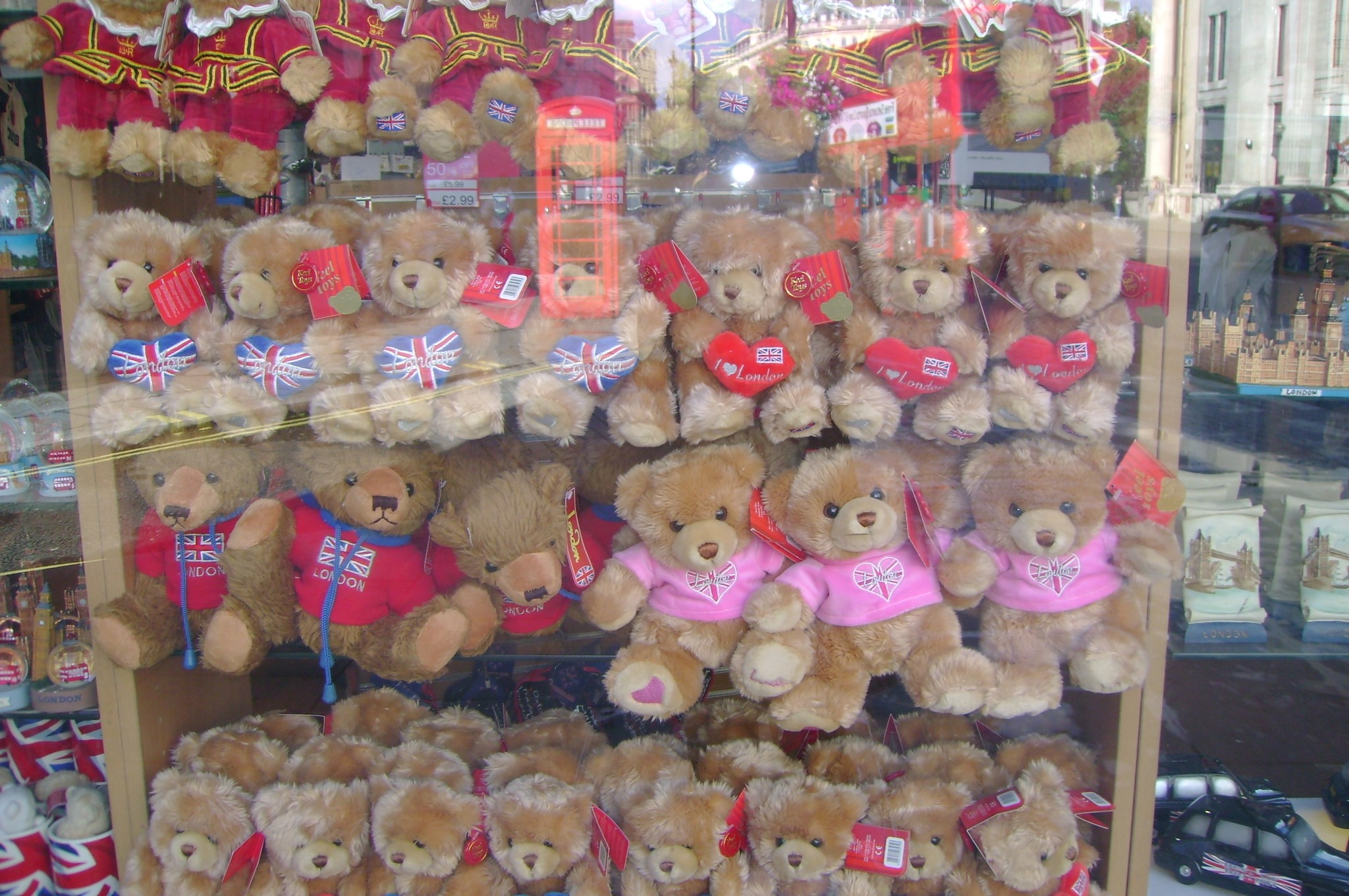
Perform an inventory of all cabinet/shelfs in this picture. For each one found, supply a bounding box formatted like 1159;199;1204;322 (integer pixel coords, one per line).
1167;107;1348;661
0;64;99;721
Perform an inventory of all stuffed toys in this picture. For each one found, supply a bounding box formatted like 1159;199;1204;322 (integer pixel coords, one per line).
226;438;463;682
570;439;817;721
940;438;1150;724
63;202;290;445
660;204;833;441
982;198;1148;435
307;207;531;448
0;0;1175;895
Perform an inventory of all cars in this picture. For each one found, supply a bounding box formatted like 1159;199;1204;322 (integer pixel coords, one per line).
1199;183;1349;271
1154;753;1286;846
1153;793;1349;895
